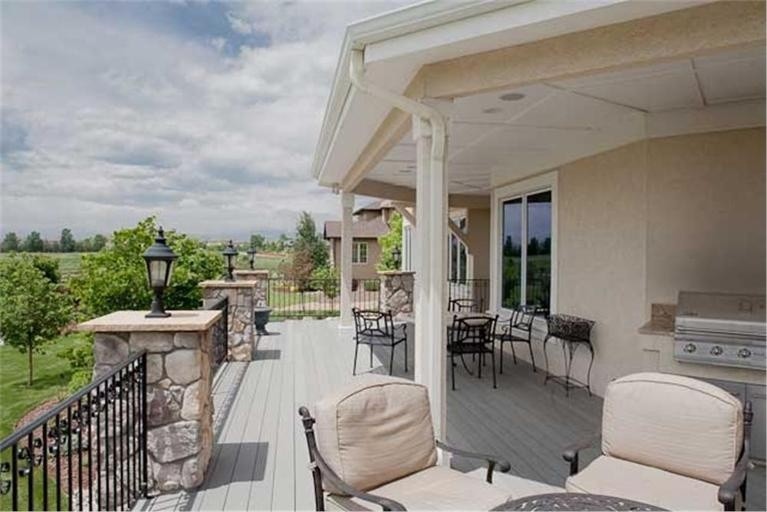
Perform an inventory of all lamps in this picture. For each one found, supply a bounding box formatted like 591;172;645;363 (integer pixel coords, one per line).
392;245;402;270
223;238;256;282
140;226;177;318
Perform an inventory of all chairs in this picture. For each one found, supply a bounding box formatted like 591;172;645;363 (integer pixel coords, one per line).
447;296;537;391
297;374;514;510
351;307;407;377
559;370;754;506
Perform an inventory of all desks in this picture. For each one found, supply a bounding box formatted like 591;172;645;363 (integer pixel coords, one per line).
491;490;669;510
